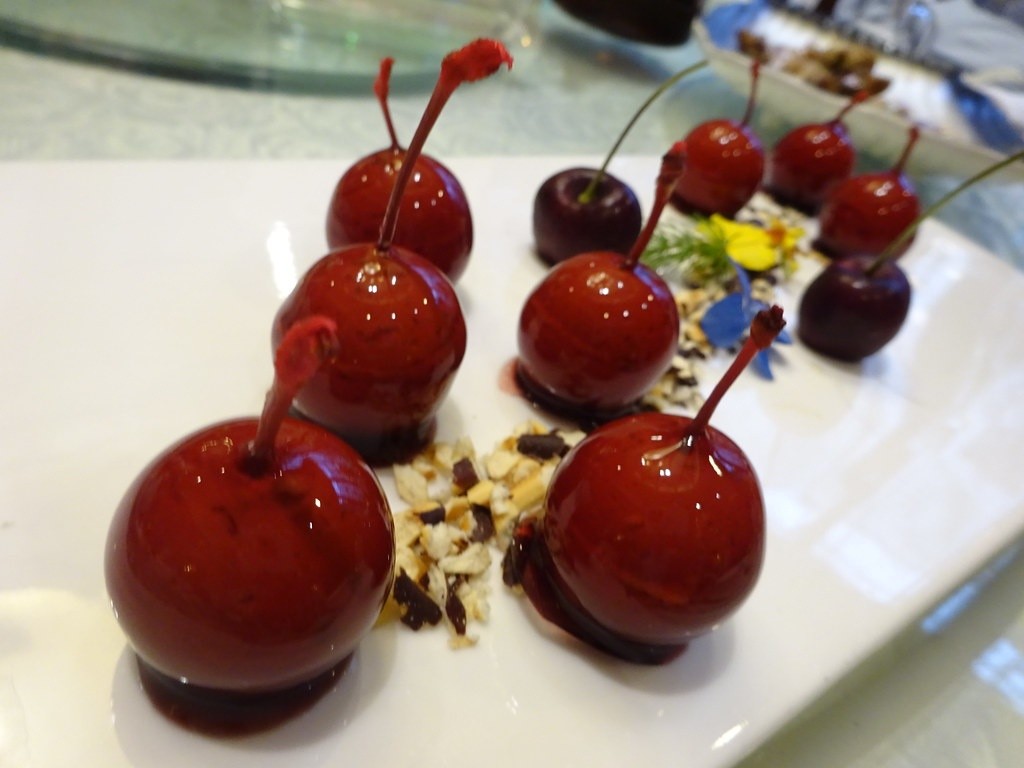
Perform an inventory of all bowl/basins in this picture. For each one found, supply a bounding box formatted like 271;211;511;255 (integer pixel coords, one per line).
692;11;1022;188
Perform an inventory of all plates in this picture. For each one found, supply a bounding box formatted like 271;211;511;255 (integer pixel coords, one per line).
3;149;1024;767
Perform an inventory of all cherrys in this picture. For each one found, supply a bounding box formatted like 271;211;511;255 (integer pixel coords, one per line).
103;29;1021;689
